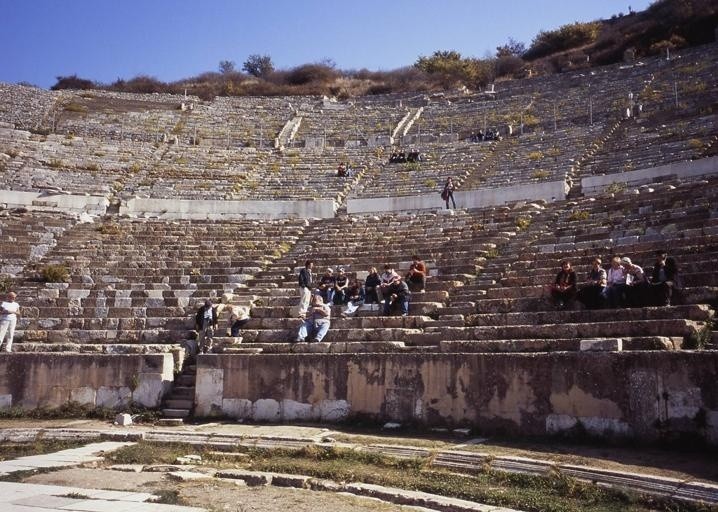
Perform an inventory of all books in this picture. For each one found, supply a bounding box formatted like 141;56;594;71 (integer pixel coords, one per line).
626;273;634;285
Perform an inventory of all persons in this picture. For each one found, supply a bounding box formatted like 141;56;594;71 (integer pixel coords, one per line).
445;177;456;209
621;257;647;309
293;254;427;343
225;304;250;337
577;257;607;310
646;249;683;306
601;256;627;308
0;292;20;353
196;300;218;354
338;161;353;177
390;148;425;162
465;127;500;142
550;260;579;310
